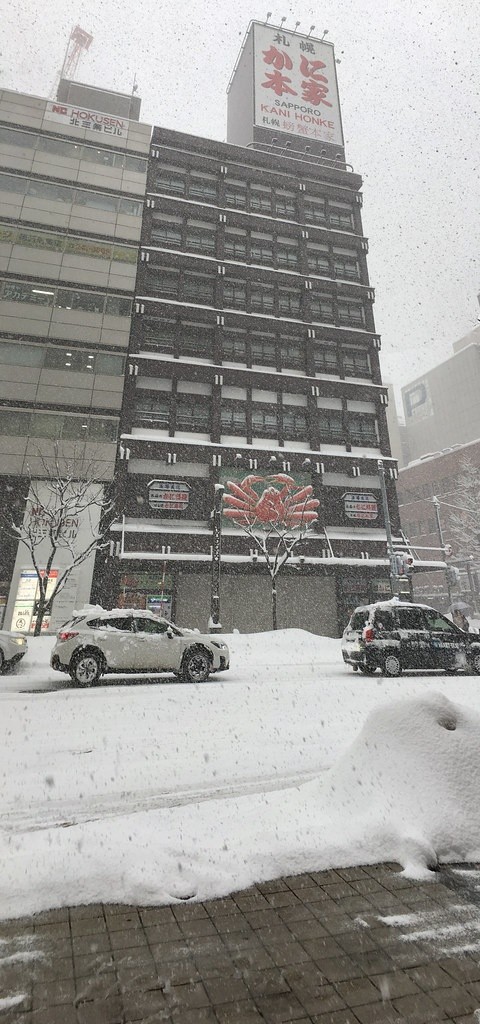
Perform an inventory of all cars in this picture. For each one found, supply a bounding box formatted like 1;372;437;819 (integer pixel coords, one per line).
0;628;28;676
342;601;480;677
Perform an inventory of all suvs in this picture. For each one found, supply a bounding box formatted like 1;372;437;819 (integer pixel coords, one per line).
49;608;231;688
